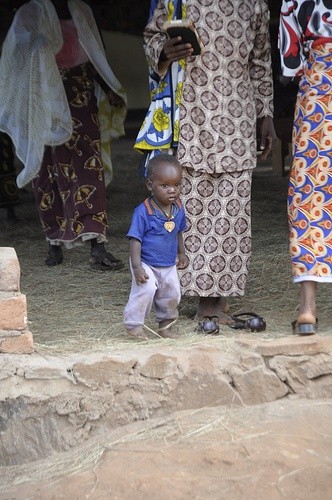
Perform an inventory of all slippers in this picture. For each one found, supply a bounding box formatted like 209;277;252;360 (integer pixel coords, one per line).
291;317;319;335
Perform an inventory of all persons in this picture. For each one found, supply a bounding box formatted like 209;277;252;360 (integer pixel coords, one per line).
122;154;189;338
278;0;332;334
0;0;125;272
144;0;277;325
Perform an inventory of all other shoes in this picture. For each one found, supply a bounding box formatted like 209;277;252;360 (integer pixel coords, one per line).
7;216;21;225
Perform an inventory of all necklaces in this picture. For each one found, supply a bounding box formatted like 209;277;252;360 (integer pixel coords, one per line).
151;196;175;233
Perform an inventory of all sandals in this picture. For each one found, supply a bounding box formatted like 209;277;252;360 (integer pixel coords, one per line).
199;316;219;334
89;252;122;270
46;248;63;265
229;312;265;331
